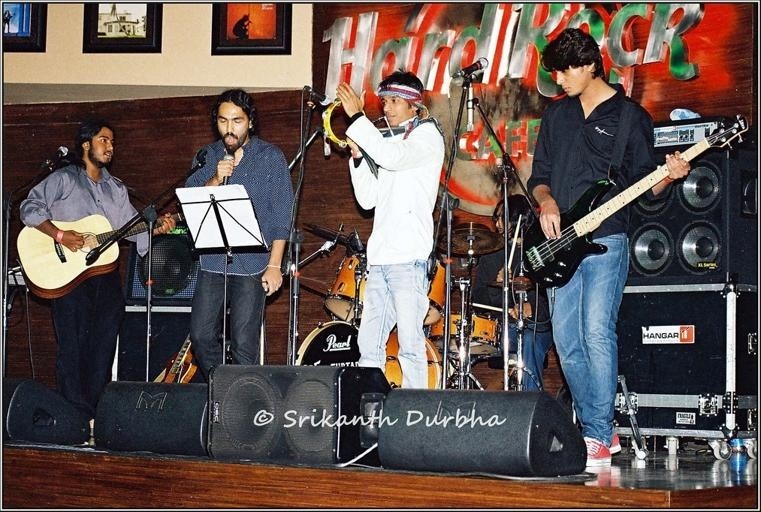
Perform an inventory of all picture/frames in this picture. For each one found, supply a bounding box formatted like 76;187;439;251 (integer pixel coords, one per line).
82;2;162;53
2;1;48;52
211;2;292;55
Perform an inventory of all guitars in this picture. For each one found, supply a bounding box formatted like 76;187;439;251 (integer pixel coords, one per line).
154;331;197;385
17;211;185;299
523;113;748;289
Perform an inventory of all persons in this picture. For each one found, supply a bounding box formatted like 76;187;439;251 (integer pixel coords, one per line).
333;70;445;389
471;192;553;393
527;27;692;469
182;86;294;382
19;116;177;454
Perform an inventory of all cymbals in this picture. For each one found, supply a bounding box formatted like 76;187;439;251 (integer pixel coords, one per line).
302;219;348;244
437;227;505;254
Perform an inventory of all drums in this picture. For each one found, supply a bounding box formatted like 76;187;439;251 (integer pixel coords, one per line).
430;308;502;352
325;254;367;320
297;318;443;389
425;260;450;328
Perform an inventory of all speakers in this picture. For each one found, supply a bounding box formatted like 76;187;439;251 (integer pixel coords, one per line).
2;377;91;446
117;235;206;382
378;388;587;477
624;115;758;284
94;380;208;457
207;365;390;468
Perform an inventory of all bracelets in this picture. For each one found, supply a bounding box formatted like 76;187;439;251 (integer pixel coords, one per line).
664;176;673;185
268;264;282;269
56;230;64;243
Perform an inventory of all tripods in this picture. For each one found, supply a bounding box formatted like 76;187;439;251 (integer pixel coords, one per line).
507;225;542;391
445;281;483;391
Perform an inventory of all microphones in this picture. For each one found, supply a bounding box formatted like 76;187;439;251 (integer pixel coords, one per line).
453;57;488;80
222;154;235;185
354;227;364;251
303;85;331;107
40;145;69;168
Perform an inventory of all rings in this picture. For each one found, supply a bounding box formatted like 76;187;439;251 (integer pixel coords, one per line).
261;281;266;283
684;161;687;166
73;247;75;250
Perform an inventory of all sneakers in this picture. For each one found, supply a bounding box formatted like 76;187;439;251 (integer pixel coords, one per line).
584;430;622;467
